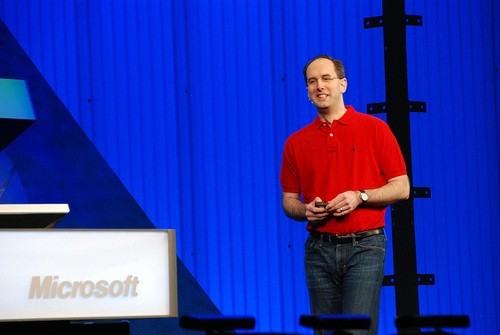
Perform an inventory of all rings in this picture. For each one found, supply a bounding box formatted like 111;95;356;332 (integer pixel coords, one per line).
340;208;342;211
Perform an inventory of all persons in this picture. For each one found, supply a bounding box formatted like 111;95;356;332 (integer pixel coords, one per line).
279;55;410;335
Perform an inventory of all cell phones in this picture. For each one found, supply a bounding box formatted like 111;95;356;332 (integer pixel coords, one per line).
314;202;328;215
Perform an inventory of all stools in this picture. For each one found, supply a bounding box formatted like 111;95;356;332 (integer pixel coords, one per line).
179;314;256;335
299;314;372;335
394;314;470;335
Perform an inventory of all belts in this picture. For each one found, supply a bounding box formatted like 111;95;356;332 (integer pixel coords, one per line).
309;229;383;243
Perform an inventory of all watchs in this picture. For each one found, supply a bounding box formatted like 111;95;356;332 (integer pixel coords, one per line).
359;189;368;205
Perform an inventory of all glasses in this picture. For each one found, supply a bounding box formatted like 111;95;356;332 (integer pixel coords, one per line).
306;75;339;85
